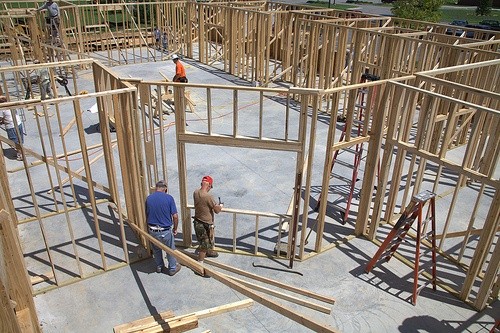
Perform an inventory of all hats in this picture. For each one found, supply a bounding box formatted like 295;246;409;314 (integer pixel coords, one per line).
171;53;180;60
202;176;213;188
156;180;167;192
0;95;7;103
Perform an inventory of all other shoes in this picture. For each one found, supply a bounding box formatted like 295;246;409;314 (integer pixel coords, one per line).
156;265;165;273
17;154;22;161
207;251;219;257
169;264;181;276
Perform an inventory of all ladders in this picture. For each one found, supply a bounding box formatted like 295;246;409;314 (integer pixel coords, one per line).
44;16;78;79
365;190;436;304
489;320;500;333
315;73;381;225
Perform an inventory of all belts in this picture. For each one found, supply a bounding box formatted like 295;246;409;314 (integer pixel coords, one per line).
147;227;169;232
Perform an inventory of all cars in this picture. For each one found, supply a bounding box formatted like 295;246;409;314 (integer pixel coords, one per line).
479;19;500;27
446;19;468;34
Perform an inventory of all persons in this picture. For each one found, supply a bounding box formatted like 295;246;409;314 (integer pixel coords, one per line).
30;0;62;37
192;175;224;278
33;58;55;106
145;182;182;276
171;54;189;83
0;96;26;160
155;24;169;51
